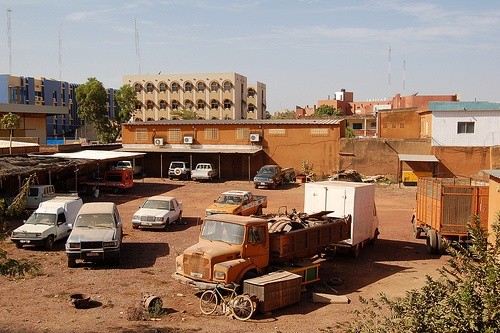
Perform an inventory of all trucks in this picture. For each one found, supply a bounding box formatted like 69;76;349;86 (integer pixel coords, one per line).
64;166;134;194
304;180;380;257
171;215;351;293
9;196;84;250
410;176;489;254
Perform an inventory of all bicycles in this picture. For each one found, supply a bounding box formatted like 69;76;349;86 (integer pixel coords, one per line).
200;281;254;321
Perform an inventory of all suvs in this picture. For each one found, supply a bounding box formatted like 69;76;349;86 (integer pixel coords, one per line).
168;161;190;180
253;165;284;190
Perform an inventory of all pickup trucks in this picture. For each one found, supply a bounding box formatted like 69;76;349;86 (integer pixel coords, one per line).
4;184;78;212
205;191;268;217
110;161;143;176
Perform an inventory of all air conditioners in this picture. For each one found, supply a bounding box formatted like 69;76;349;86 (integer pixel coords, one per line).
249;133;259;142
184;136;193;144
154;138;163;146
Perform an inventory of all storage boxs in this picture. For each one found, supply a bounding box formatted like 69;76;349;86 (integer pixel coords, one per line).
243;271;303;313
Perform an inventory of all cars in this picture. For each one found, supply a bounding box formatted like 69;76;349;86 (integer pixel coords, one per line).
191;163;219;181
131;196;182;231
65;202;123;267
